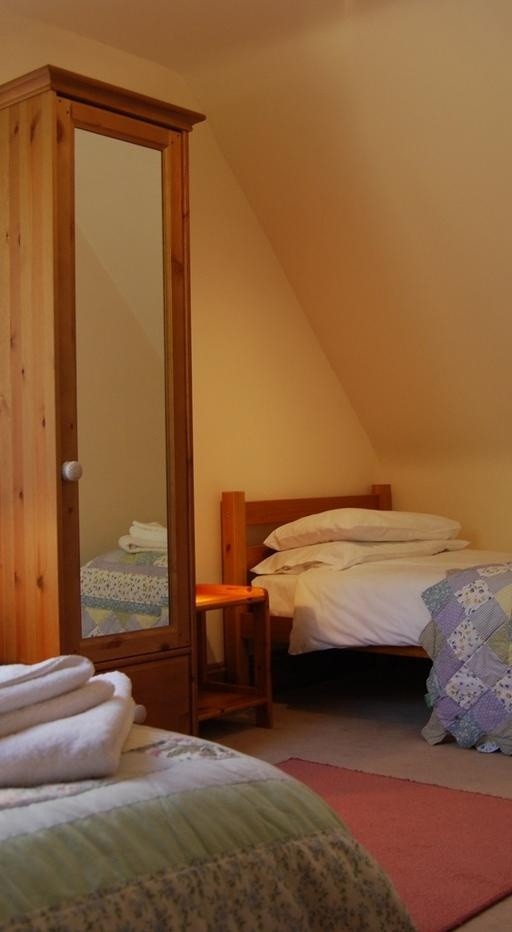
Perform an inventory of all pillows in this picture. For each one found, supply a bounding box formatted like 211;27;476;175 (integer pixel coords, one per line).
249;537;471;576
263;503;461;553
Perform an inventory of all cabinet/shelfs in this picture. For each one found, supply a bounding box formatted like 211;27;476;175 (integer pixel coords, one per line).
1;64;208;737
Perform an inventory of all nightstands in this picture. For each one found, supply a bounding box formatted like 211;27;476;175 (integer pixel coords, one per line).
197;584;275;732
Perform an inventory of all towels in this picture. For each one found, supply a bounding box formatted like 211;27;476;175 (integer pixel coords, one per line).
1;654;135;787
118;520;168;553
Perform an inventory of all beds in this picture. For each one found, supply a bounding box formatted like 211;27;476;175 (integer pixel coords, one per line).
81;547;171;640
222;481;511;758
1;715;414;931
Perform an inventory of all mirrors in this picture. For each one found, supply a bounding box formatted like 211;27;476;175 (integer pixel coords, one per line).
72;122;173;641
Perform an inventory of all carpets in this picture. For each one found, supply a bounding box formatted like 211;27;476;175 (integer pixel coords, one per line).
268;758;511;930
